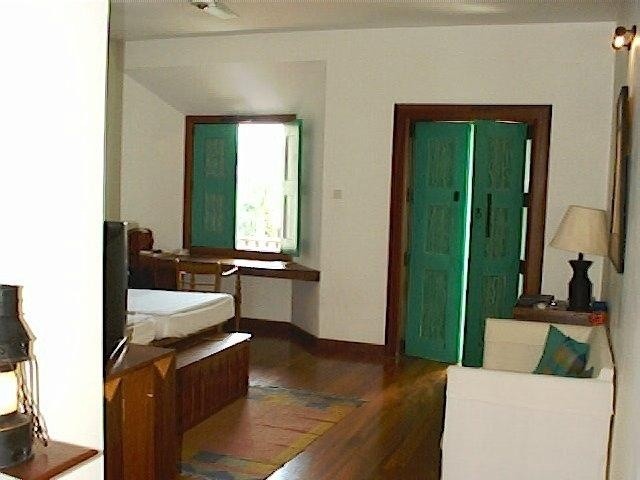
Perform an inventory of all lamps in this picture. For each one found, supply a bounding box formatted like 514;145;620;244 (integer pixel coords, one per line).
549;205;612;311
612;24;636;51
0;284;48;472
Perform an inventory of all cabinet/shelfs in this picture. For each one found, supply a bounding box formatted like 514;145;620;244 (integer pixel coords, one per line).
176;332;251;434
105;343;180;480
514;294;610;325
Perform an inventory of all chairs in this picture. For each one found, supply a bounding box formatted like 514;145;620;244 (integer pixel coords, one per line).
174;256;241;333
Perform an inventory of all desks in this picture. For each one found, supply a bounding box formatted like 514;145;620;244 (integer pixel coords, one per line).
155;254;319;281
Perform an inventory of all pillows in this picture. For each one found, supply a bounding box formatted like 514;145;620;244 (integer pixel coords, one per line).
532;324;590;378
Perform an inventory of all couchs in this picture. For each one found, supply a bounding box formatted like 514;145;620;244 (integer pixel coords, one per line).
440;317;614;480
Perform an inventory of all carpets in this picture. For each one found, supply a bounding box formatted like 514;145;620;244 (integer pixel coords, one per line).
180;383;365;480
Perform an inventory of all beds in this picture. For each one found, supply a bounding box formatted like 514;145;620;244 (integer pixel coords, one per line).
127;288;236;353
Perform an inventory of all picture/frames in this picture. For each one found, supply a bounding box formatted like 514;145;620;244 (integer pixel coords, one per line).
609;85;628;273
